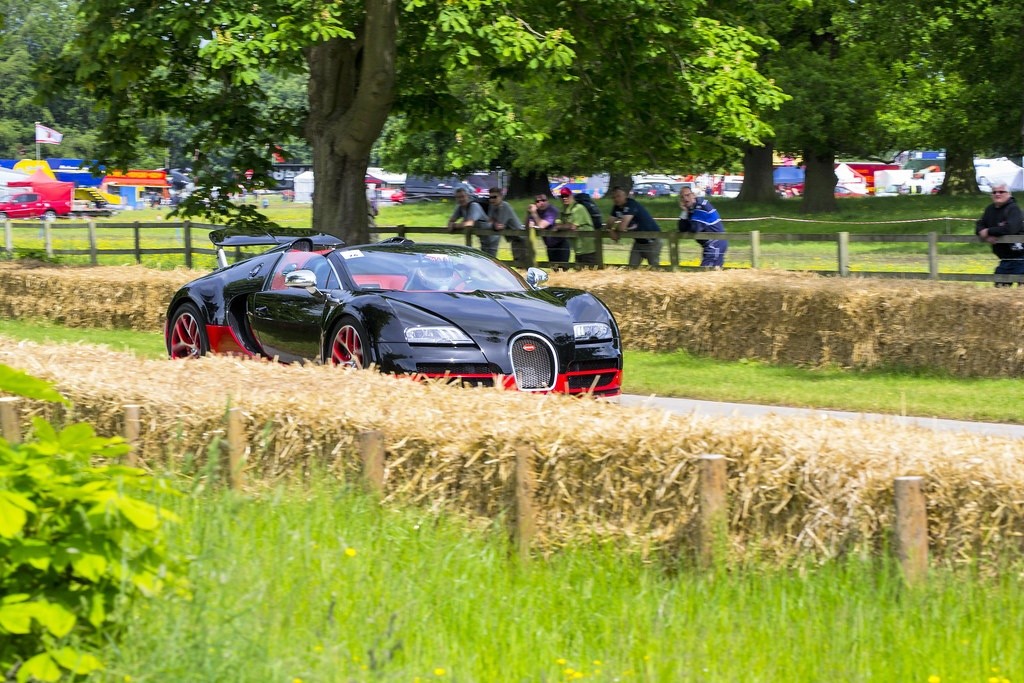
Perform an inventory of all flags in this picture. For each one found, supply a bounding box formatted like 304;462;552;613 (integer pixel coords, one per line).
36;125;63;145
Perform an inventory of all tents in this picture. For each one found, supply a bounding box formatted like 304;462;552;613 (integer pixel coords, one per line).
773;150;1024;196
293;168;408;204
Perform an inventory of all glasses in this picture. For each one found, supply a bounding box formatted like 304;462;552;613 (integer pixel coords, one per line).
489;195;501;199
560;194;570;199
992;191;1009;194
536;199;547;203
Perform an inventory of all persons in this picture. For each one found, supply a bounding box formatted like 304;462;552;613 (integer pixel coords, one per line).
976;182;1024;286
678;186;727;266
448;188;500;256
486;187;534;261
554;187;596;263
528;193;570;262
609;189;663;265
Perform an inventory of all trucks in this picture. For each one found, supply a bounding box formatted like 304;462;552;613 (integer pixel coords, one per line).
7;182;113;216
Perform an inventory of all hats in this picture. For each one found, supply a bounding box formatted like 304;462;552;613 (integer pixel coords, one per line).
560;187;572;195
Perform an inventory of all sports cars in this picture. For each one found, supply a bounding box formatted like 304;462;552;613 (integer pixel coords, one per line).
164;229;622;396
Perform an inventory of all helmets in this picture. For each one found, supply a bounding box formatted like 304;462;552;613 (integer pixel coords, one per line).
417;253;453;290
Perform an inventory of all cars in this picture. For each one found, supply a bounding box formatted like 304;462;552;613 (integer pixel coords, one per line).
834;186;867;197
0;192;57;221
629;182;678;196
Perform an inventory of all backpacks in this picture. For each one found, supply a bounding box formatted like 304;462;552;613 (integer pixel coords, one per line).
466;198;489;222
570;193;603;229
